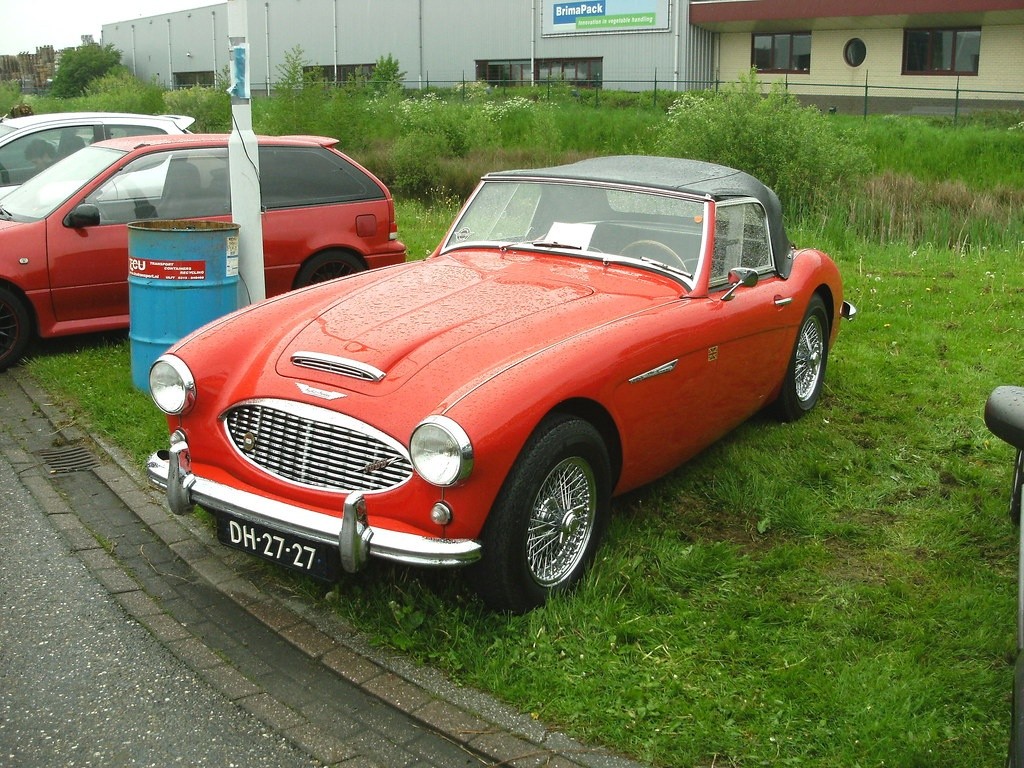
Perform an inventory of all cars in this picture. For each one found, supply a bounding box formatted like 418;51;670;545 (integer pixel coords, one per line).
143;156;856;614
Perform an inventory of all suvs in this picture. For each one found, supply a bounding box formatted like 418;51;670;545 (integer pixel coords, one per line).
0;110;229;216
0;134;408;376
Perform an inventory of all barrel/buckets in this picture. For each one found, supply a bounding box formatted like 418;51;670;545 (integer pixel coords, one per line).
128;219;241;394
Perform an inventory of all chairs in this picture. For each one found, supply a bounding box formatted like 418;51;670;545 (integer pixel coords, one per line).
60;137;85;156
152;162;231;216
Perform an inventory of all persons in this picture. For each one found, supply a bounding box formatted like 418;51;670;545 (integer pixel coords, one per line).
24;138;64;179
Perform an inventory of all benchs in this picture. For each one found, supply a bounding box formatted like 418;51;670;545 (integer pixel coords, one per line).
573;211;774;291
